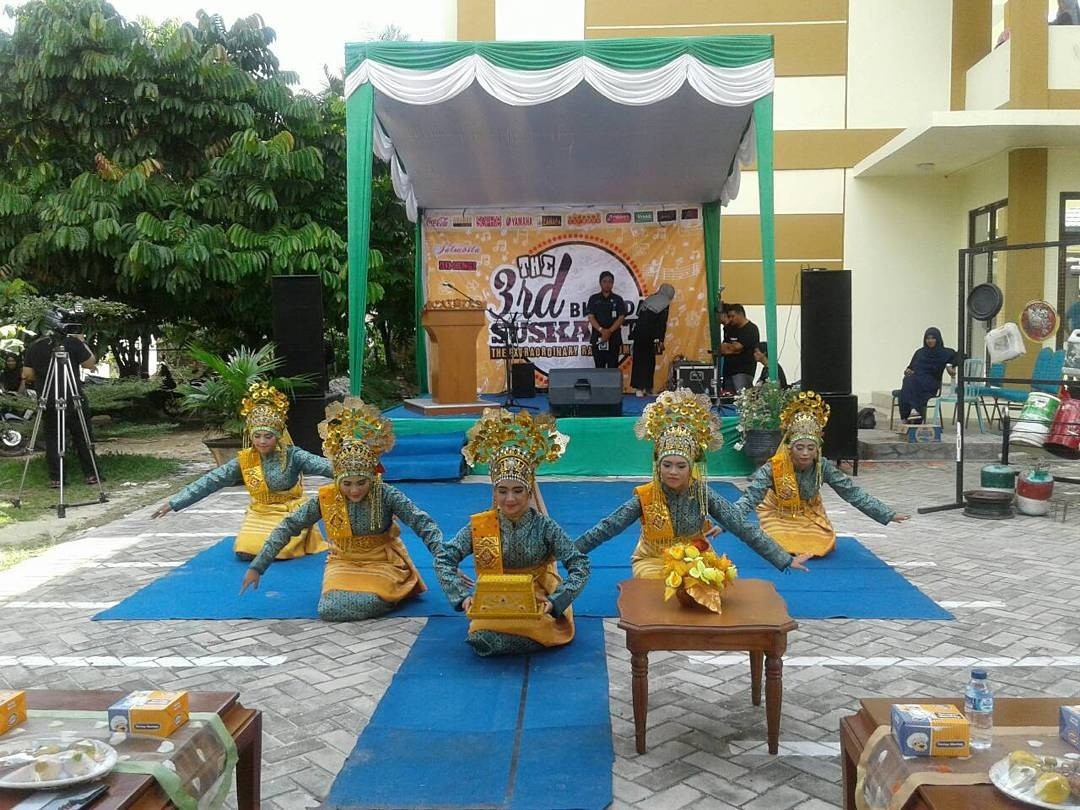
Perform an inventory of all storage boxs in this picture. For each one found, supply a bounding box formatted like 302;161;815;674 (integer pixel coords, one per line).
896;425;944;443
107;690;189;736
673;366;717;397
889;703;970;756
1058;705;1080;751
0;690;27;735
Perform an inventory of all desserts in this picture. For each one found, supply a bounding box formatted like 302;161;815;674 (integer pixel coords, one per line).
1034;772;1071;803
1009;751;1043;778
67;739;95;764
32;758;64;780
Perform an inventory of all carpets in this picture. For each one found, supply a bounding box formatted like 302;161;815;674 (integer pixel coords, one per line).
91;480;958;810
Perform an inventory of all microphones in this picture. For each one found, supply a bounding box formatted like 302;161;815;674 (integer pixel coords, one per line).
719;285;725;291
442;282;450;286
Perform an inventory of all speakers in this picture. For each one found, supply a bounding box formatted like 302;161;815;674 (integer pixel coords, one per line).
799;270;859;461
512;363;536;399
273;273;342;459
548;367;622;419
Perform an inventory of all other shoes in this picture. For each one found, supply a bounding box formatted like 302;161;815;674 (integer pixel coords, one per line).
87;474;106;484
51;479;69;487
908;413;921;421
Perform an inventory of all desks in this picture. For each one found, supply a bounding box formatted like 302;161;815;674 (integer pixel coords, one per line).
0;687;262;810
839;697;1080;810
615;579;798;758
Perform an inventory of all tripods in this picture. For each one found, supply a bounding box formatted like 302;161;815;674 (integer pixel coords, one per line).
11;342;111;518
448;284;540;411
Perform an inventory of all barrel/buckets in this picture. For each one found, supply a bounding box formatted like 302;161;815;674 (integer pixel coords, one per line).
984;323;1026;365
1043;397;1080;459
1061;328;1080;376
1008;391;1061;447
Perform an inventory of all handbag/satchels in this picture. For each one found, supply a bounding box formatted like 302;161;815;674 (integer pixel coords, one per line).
856;408;876;430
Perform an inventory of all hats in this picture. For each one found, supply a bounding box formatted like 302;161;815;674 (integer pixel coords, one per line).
633;388;723;522
461;406;570;494
317;397;395;533
778;390;831;488
241;382;290;470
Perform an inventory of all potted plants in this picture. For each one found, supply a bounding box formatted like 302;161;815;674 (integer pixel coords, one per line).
178;338;323;466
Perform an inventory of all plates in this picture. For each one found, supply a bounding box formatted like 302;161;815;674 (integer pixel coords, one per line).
0;738;118;789
989;754;1080;810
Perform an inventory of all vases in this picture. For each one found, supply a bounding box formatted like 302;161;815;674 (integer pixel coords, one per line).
744;428;782;470
675;583;699;607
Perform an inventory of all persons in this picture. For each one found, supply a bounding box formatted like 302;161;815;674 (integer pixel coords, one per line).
704;390;911;559
151;383;336;560
630;284;675;397
898;327;955;422
0;353;27;395
24;332;106;489
587;271;625;368
714;303;760;391
239;398;474;624
754;342;786;388
434;408;590;659
572;388;814;581
1066;290;1080;335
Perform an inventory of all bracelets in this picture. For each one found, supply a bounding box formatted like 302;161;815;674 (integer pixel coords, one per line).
785;558;793;575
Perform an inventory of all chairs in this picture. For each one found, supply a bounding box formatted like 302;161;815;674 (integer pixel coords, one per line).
889;347;1066;435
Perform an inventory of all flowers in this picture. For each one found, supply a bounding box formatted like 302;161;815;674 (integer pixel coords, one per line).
733;375;799;451
654;543;739;613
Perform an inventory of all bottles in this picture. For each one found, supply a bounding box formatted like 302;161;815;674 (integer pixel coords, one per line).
963;668;994;749
